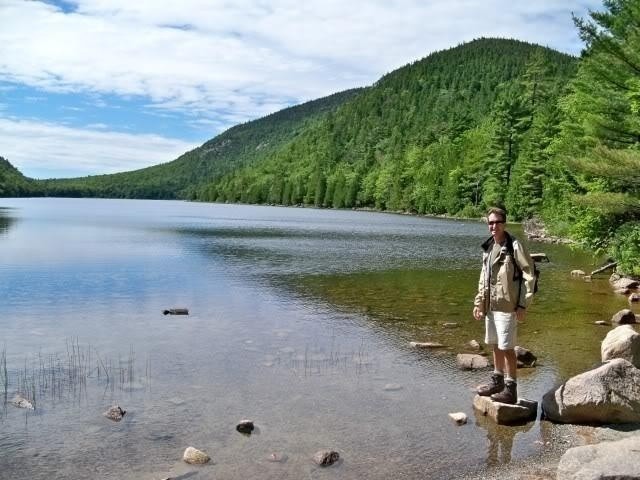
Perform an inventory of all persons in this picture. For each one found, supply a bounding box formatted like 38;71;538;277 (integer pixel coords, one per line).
472;207;536;405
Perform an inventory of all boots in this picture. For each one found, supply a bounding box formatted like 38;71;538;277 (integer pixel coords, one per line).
491;381;517;403
478;374;504;395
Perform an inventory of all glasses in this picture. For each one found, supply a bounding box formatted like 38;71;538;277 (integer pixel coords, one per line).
489;221;503;225
